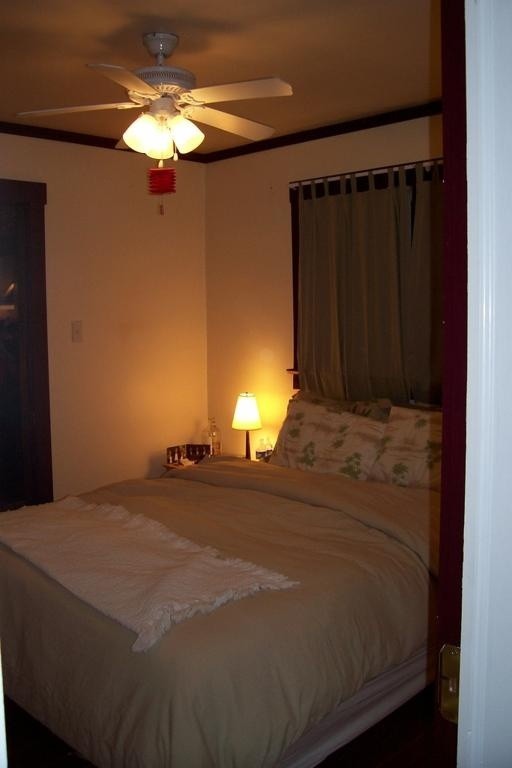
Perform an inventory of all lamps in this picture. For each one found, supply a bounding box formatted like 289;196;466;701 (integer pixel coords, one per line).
231;393;263;459
122;97;206;168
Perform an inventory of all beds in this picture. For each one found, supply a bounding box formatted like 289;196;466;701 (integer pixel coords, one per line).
0;456;440;768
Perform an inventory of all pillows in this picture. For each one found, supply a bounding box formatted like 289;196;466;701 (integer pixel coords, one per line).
265;390;442;491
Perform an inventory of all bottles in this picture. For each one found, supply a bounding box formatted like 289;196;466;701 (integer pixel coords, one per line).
202;416;221;463
256;436;274;461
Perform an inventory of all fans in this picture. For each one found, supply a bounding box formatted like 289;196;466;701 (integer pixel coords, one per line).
16;31;295;151
